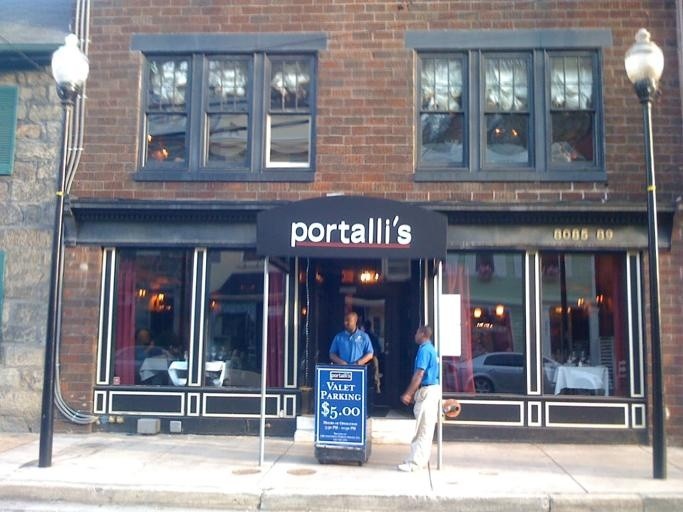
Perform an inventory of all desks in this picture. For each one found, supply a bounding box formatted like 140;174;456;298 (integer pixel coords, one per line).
139;355;231;387
552;366;610;397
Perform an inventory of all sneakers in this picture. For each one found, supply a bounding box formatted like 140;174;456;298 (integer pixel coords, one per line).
398;459;421;472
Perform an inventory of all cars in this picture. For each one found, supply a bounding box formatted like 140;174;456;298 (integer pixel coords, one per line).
114;345;175;380
447;352;561;398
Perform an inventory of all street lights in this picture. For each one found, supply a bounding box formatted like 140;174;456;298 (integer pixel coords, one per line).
37;30;90;465
624;28;668;477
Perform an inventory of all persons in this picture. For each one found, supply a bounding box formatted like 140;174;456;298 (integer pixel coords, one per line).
493;333;508;352
328;310;382;419
398;325;441;474
135;328;164;384
